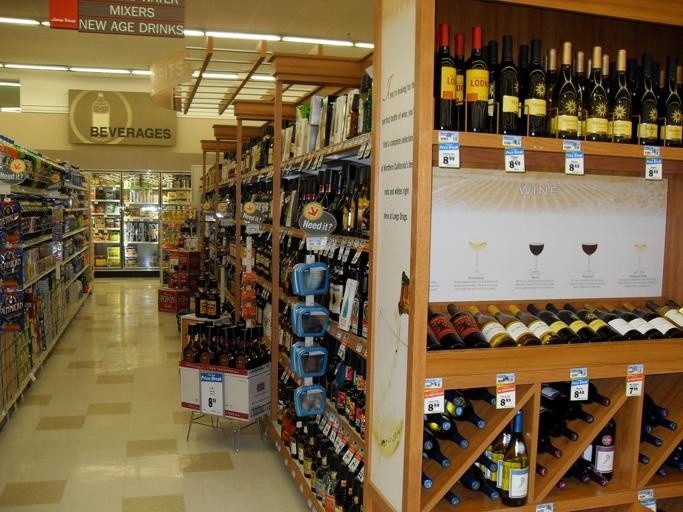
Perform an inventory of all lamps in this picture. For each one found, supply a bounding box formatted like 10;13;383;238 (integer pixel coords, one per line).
0;82;21;87
41;22;50;26
184;29;204;38
355;43;375;49
283;36;353;48
251;75;276;81
206;31;281;42
0;107;20;113
132;70;152;76
193;72;240;79
0;17;39;25
69;67;130;74
5;63;70;71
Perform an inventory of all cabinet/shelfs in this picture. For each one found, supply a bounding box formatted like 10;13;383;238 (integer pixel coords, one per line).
364;0;682;510
81;171;191;277
0;134;95;424
200;52;373;511
160;206;196;286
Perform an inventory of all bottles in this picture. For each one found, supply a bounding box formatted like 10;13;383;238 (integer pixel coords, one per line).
240;128;275;175
420;471;432;489
495;33;520;135
557;479;567;489
539;406;578;442
201;191;218;217
290;418;303;459
460;472;479;491
433;23;454;131
527;304;584;343
224;261;236;297
303;437;316;479
485;40;499;134
601;55;611;97
516;45;529;83
195;280;207;318
276;304;299;357
583;47;609;142
207;280;220;318
488;305;541;346
468;305;515;347
444;490;460;506
199;164;222;188
584;304;644;340
277;390;287;424
657;66;665;106
640;432;662;447
572;463;590;483
238;324;246;333
334;479;347;512
623;302;683;338
545;304;603;343
665;300;683;314
592;417;616;481
666;446;683;469
638;453;649;465
327;243;369;338
201;326;216;366
603;302;664;339
585;60;592;84
608;49;633;144
203;219;217;243
643;393;669;416
443;391;486;429
637;53;658;146
463;26;489;133
521;38;546;137
467;465;500;501
213;324;222;354
423;411;470;449
509;304;561;345
247;328;266;365
279;236;326;307
573;52;583;141
284;403;297;446
540;386;594;423
554;42;578;140
652;415;677;430
545;49;558;138
447;304;492;348
206;320;213;326
491;423;513;495
351;487;364;512
197;321;205;350
230;326;237;350
241;229;273;282
537;429;561;458
428;308;467;349
325;472;337;512
453;32;465;132
208;246;220;279
642;409;656;421
564;304;622;342
580;444;592;462
459;387;500;406
477;454;498;471
501;411;529;506
218;185;235;219
479;445;492;487
215;328;235;368
313;333;365;439
218;224;236;258
315;453;331;501
426;324;444;350
675;67;683;99
536;464;546;477
251;286;271;338
311;449;324;492
423;428;450;469
256;326;271;363
578;458;607;487
659;56;683;147
236;331;253;369
646;300;683;332
221;150;237;180
276;366;277;384
297;425;309;464
242;164;371;240
657;466;665;476
343;483;352;512
627;58;638;108
282;73;373;160
183;323;199;363
280;398;292;441
550;379;611;406
641;422;652;434
